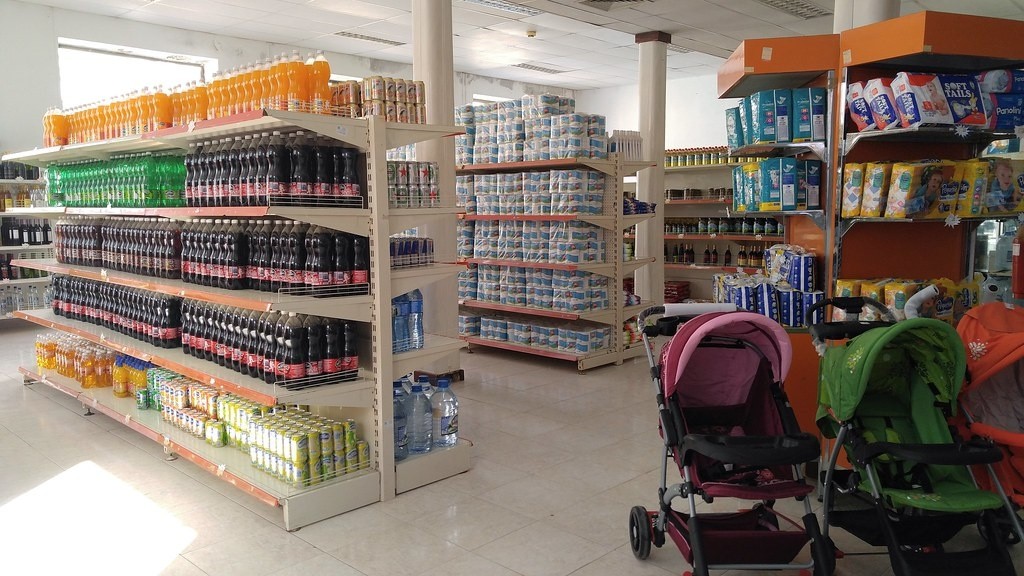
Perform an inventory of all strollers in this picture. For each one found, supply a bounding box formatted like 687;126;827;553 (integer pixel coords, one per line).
804;293;1019;576
628;300;837;576
904;282;1024;548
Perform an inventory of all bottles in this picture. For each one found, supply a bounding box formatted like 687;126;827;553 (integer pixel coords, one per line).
35;49;370;404
630;225;635;235
763;241;782;269
711;244;718;266
748;246;754;267
407;289;424;349
391;371;460;459
679;242;684;264
724;244;731;267
673;242;678;264
394;293;411;353
738;244;742;266
740;245;747;266
391;299;398;356
976;217;1021;303
757;246;762;267
664;242;669;264
689;243;695;264
751;246;758;268
684;244;690;265
663;146;784;238
0;152;55;316
703;244;710;266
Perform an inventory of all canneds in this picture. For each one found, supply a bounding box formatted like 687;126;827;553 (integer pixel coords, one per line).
136;368;370;488
327;76;428;125
384;236;436;271
388;160;441;211
664;188;784;236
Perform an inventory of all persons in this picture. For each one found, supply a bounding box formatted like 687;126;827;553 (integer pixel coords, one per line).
989;163;1021;214
926;80;950;116
916;295;937;317
912;169;944;213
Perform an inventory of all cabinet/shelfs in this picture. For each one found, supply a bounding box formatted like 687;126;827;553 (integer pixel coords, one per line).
1;53;1023;531
